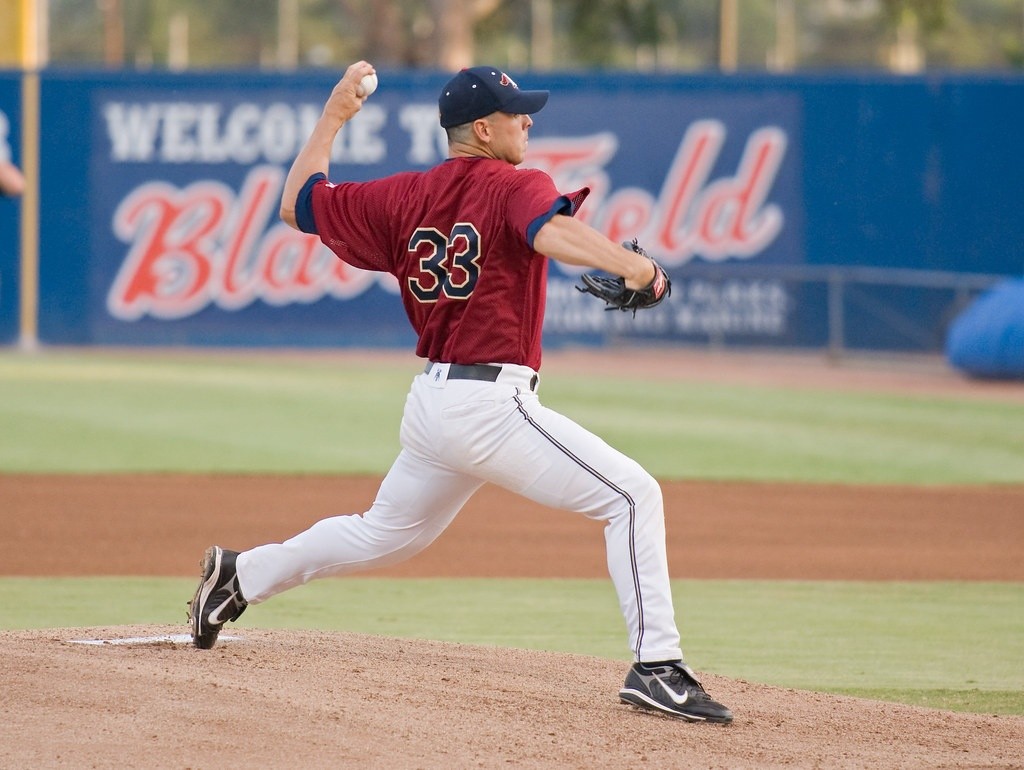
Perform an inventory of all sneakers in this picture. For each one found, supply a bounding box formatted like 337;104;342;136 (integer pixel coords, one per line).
188;546;248;650
618;661;734;722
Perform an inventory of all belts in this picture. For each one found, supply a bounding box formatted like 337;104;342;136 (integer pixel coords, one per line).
424;360;537;391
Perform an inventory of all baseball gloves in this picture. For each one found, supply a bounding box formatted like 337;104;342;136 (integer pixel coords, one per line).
573;236;672;320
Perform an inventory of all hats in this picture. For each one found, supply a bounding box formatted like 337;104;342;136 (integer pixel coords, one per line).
440;66;551;128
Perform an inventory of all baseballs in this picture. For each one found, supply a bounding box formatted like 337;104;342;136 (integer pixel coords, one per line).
356;71;379;98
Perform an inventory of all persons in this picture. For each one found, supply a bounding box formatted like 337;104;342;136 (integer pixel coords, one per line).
187;61;733;724
